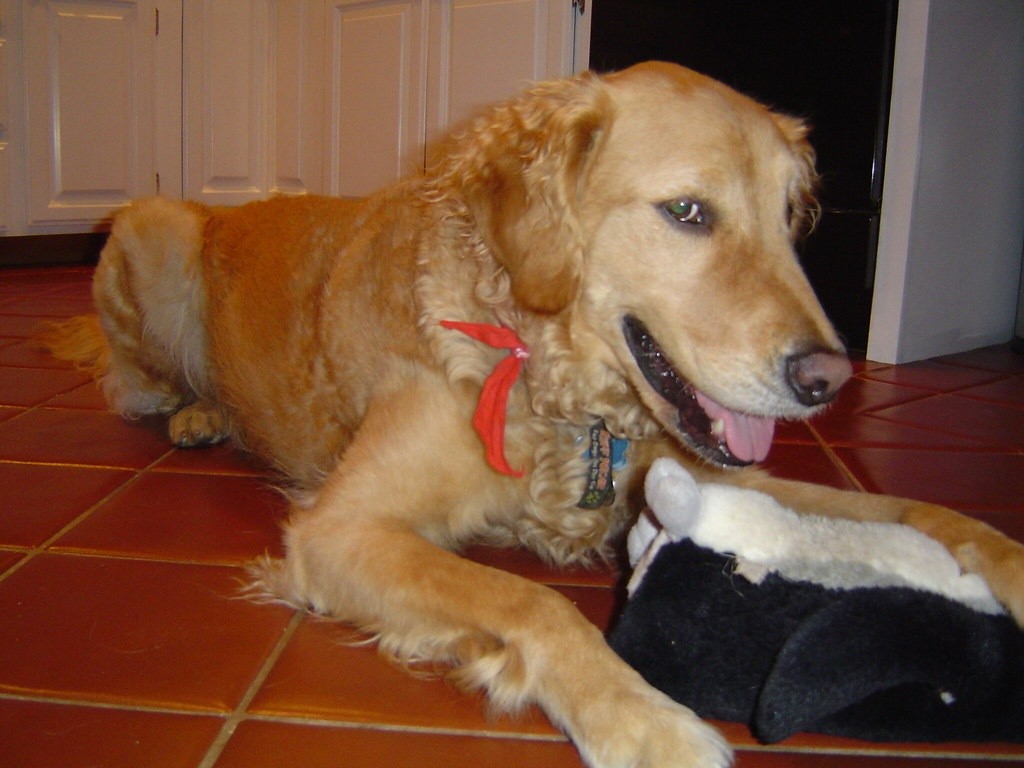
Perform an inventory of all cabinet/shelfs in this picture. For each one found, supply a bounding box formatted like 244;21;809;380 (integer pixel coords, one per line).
0;0;593;236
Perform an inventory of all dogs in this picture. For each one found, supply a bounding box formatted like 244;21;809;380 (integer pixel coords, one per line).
47;58;1024;768
